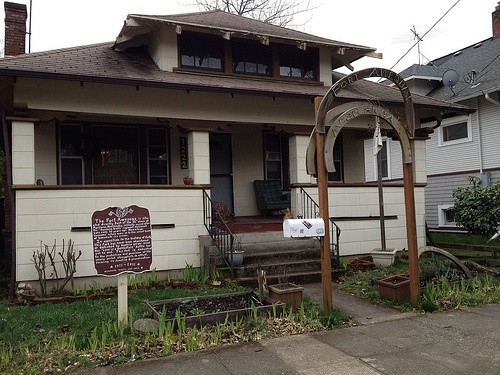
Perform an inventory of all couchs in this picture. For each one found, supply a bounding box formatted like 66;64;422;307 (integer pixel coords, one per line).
254;178;291;217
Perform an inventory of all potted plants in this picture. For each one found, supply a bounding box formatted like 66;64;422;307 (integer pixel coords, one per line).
228;233;245;267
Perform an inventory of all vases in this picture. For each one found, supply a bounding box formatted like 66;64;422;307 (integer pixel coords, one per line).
371;248;396;268
269;283;304;309
377;274;411;304
184;177;193;185
